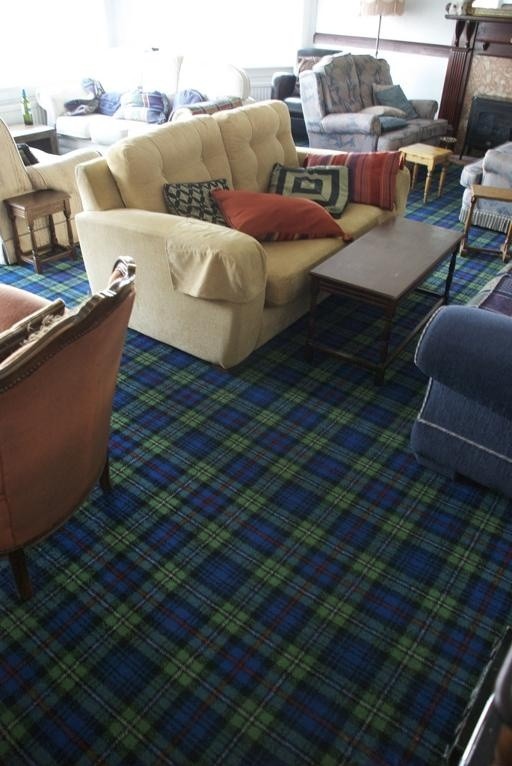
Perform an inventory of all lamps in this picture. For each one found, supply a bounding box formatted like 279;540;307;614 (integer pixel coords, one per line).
360;0;406;59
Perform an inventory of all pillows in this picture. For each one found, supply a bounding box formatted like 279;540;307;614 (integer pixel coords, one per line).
378;115;411;132
375;84;419;119
291;55;323;97
265;161;352;217
209;187;354;243
372;82;395;105
357;104;408;118
304;148;408;213
161;177;233;228
63;77;210;124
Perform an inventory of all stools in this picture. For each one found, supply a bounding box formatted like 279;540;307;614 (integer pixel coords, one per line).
397;142;454;203
460;183;512;263
2;188;76;275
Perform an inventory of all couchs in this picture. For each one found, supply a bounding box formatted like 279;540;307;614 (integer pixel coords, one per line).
459;139;512;235
32;45;251;156
270;48;344;141
74;97;414;368
0;115;104;266
298;51;449;170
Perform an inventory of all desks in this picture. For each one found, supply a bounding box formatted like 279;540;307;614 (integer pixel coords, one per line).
6;122;60;154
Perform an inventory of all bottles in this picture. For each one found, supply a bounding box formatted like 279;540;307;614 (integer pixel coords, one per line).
19;89;33;126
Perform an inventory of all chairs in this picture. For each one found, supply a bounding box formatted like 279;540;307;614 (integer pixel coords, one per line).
0;251;138;602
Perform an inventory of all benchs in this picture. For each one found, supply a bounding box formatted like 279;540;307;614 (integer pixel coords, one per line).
411;254;512;500
